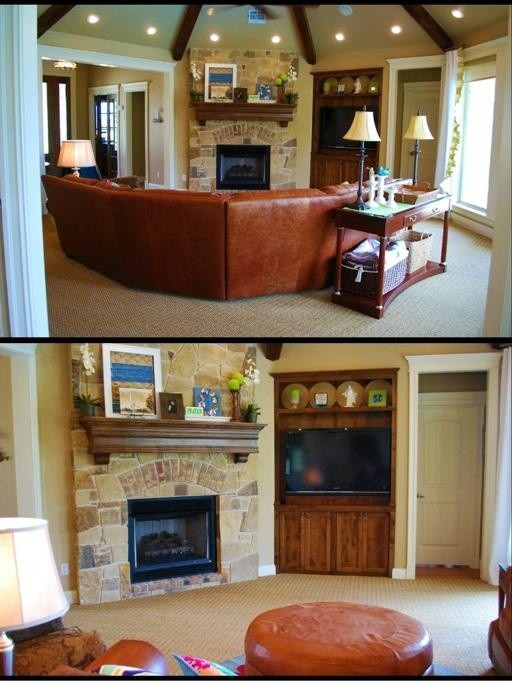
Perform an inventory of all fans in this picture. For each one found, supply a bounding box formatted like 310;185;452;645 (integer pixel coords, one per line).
207;5;320;22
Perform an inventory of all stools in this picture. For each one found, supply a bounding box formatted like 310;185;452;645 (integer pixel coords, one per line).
237;604;435;677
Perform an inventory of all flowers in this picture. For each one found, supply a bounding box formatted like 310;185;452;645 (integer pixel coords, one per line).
287;64;297;95
243;357;262;415
78;344;102;403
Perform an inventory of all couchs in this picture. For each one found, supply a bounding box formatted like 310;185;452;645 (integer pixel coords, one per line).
6;630;104;677
41;173;405;301
48;639;170;677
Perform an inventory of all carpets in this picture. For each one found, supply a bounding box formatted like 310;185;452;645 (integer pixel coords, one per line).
222;653;463;677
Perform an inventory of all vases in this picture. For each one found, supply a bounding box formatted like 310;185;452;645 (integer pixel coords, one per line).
277;85;284;103
231;390;241;420
286;96;296;104
82;404;95;417
245;412;258;422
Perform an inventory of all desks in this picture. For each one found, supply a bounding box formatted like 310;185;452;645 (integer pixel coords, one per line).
488;561;512;676
329;191;453;319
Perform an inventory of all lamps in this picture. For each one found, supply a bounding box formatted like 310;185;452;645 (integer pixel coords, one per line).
0;516;69;647
57;139;96;175
404;112;436;186
343;105;380;210
51;61;78;72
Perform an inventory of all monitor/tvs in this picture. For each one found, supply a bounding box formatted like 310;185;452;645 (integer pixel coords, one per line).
320;105;378;150
281;427;392;496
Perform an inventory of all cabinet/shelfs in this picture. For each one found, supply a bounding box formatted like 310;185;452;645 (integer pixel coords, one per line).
274;510;333;576
309;153;343;189
333;510;393;577
344;154;375;183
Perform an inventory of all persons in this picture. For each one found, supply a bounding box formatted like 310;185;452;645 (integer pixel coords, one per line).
340;385;358;407
352;78;362;93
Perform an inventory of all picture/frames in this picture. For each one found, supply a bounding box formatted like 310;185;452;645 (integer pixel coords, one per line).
160;391;186;421
234;86;248;103
204;63;237;103
101;343;165;419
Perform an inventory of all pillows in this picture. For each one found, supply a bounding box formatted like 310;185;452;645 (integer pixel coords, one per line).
172;652;240;677
64;176;188;191
